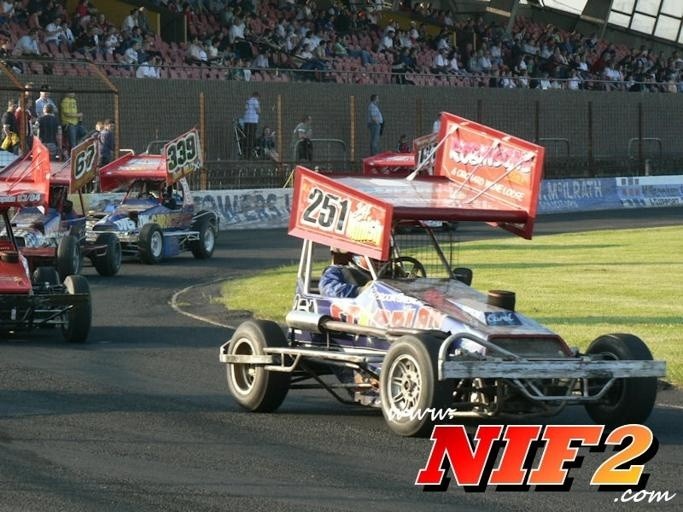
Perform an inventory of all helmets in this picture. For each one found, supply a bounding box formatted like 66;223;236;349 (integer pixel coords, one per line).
352;233;395;278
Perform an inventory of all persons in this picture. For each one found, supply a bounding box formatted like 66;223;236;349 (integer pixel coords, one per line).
45;186;72;221
317;227;421;299
159;184;177;210
0;0;683;192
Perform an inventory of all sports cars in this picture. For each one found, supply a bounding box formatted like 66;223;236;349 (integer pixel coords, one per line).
362;132;459;235
0;128;220;341
219;111;667;438
